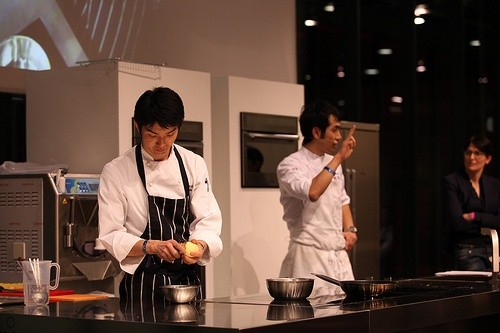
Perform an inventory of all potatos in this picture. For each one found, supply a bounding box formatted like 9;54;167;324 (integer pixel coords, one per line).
182;241;199;258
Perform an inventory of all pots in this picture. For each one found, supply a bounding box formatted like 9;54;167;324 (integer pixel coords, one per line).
311;271;398;300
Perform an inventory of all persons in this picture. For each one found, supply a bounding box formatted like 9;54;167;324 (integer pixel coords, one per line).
98;87;222;298
246;145;267;186
276;102;359;284
446;135;500;272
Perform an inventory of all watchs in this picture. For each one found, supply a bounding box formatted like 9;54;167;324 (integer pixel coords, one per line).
343;226;358;234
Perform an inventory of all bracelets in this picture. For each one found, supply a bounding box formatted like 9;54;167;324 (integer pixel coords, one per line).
142;240;149;255
323;167;336;175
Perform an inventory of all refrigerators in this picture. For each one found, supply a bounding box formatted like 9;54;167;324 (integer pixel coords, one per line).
331;121;382;280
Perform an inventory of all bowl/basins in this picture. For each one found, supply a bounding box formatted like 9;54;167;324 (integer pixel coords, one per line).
265;299;315;321
163;303;200;322
265;278;314;300
159;285;201;304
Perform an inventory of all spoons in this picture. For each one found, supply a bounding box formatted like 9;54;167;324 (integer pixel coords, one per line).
29;257;48;305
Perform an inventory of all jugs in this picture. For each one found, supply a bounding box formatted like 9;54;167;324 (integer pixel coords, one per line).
16;260;60;306
